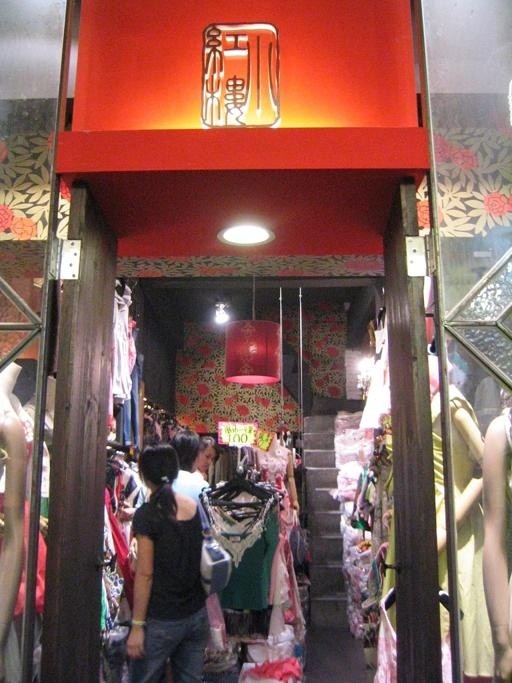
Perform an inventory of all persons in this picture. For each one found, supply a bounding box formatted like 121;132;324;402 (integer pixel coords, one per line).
0;361;57;683
252;432;300;528
382;382;512;683
125;427;218;683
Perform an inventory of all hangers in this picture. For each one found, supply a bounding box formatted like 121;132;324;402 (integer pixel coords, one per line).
105;443;136;475
201;477;285;510
143;397;174;425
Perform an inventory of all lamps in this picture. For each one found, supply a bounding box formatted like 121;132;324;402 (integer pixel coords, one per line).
223;276;280;384
215;225;277;247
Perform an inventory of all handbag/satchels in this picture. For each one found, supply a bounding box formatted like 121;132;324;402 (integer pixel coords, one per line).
290;527;305;569
200;536;233;596
